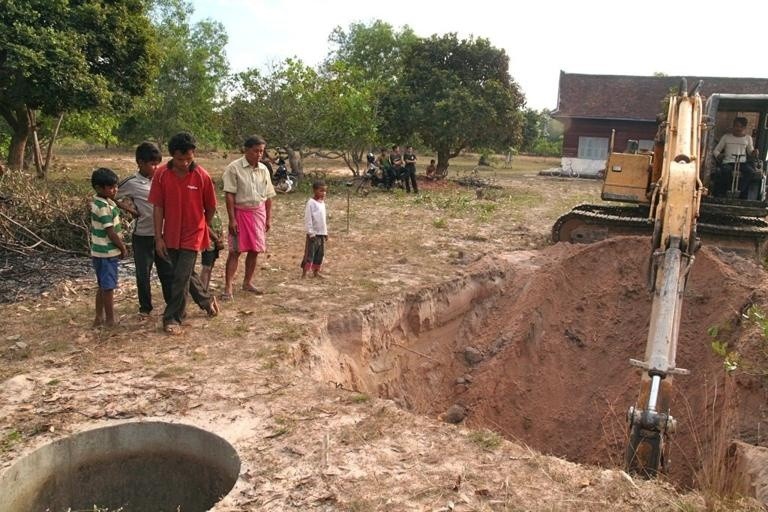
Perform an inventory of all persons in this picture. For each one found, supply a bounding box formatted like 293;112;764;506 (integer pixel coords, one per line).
375;146;418;193
116;142;187;321
713;118;759;200
426;159;436;180
201;209;224;294
221;134;277;301
260;149;278;177
88;167;126;327
301;181;328;280
149;134;219;336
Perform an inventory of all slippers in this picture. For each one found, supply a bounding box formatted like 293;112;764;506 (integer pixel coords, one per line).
208;296;219;317
164;324;180;335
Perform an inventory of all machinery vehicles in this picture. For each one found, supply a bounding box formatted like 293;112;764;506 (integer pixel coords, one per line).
550;77;767;478
